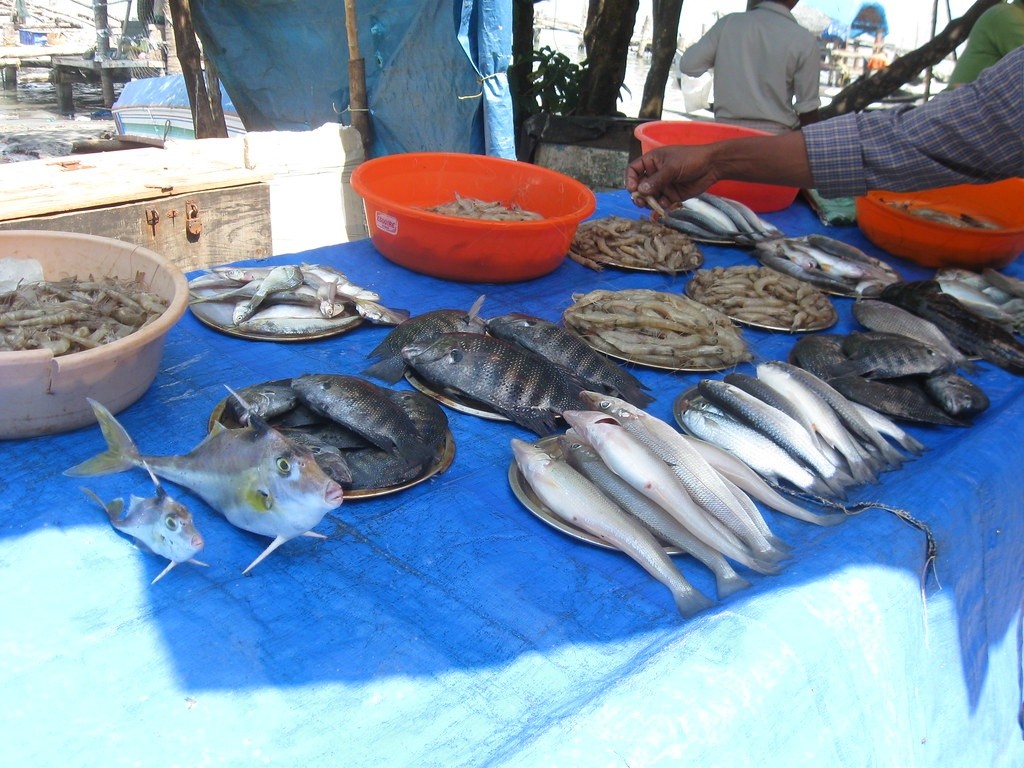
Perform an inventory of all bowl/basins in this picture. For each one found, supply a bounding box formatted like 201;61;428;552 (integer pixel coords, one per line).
856;176;1024;271
1;230;189;439
349;151;596;281
634;119;799;212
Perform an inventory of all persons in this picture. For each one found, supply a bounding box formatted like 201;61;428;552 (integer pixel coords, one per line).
625;43;1024;211
948;0;1024;92
679;0;821;134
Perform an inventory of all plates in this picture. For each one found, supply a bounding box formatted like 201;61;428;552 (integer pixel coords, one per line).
649;207;784;244
508;432;685;555
189;274;362;342
209;397;455;499
572;219;704;272
758;237;904;298
405;370;619;421
562;314;737;371
672;386;706;440
685;278;839;331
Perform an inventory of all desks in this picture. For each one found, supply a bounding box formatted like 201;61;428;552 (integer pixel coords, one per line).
0;187;1023;768
52;55;166;117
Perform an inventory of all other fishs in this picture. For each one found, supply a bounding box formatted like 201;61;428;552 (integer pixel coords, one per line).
61;192;1024;622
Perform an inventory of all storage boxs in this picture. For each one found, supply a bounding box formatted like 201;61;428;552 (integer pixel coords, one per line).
18;29;49;46
0;178;273;274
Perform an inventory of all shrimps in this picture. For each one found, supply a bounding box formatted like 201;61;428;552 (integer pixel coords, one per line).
0;270;168;357
